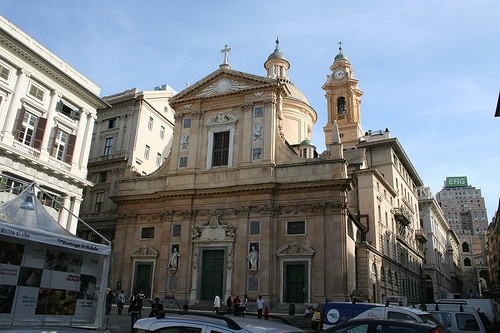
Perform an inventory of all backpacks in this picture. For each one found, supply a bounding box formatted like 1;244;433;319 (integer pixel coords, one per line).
131;301;140;312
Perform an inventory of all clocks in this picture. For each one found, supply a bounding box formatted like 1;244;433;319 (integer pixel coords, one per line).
333;69;346;81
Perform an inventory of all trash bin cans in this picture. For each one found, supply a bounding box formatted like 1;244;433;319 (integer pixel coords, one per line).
289;302;295;315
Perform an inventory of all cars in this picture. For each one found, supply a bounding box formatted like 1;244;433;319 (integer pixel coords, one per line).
319;317;439;333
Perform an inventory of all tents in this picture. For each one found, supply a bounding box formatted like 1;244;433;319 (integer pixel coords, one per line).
0;183;112;333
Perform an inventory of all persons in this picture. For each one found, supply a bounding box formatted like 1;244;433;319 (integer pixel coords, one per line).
127;288;143;333
256;295;264;319
247;245;260;271
385;297;432;312
305;306;315;318
149;297;164;319
117;289;125;315
214;293;248;315
170;248;181;268
105;290;115;315
264;307;269;320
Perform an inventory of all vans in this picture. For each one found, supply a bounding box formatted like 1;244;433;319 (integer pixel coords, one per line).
312;296;498;333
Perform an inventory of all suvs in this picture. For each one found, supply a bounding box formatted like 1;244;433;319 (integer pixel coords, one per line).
130;310;308;333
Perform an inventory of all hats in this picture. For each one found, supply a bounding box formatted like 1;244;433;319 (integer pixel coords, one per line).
420;303;427;308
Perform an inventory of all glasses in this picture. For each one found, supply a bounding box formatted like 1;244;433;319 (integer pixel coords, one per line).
154;300;157;301
421;309;427;311
